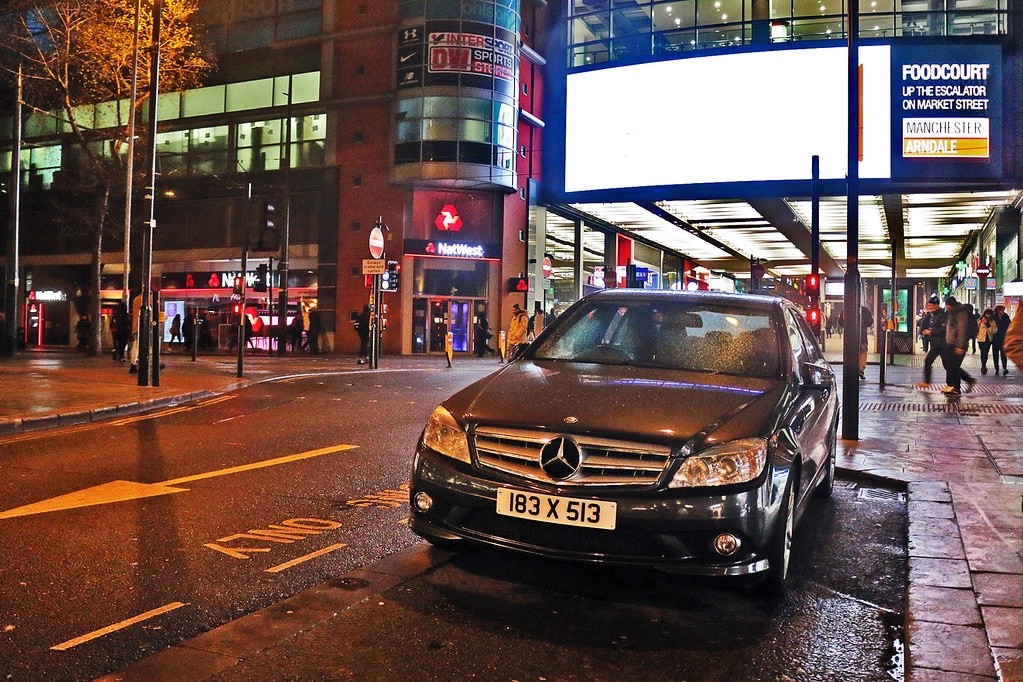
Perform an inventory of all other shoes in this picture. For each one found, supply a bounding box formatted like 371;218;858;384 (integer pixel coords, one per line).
129;363;138;374
994;369;1000;374
476;354;483;357
366;359;369;363
941;386;954;393
358;359;364;364
1003;369;1008;375
168;348;172;351
917;381;930;387
859;371;865;379
160;364;165;371
981;367;987;374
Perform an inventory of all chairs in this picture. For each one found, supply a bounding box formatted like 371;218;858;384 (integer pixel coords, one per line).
625;321;778;376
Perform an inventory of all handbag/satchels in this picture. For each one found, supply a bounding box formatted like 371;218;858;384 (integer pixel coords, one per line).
484;330;493;338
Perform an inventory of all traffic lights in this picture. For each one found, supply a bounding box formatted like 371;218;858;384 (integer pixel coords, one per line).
231;303;242;315
249;192;276;231
806;308;819;324
806;274;820;296
388;260;400;292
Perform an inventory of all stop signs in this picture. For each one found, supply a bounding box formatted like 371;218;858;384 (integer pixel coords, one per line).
542;257;551;278
370;227;384;259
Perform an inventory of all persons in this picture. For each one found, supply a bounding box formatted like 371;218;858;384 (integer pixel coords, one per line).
228;314;255;351
977;306;1011;376
353;304;371;365
823;310;845;339
507;303;529;359
839;305;874;380
912;296;981;397
1003;299;1023;371
289;309;325;355
527;308;557;339
474;313;497;358
110;302;133;363
169;314;182;345
73;313;92;352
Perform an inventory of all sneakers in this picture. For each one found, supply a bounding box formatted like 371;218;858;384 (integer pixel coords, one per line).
967;378;976;392
944;388;962;397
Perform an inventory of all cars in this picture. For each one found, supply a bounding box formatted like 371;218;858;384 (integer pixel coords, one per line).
409;289;840;595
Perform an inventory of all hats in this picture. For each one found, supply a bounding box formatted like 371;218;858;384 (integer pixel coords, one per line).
929;296;940;304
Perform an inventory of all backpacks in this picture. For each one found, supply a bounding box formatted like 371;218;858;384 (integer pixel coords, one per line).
955;308;978;339
519;315;531;336
